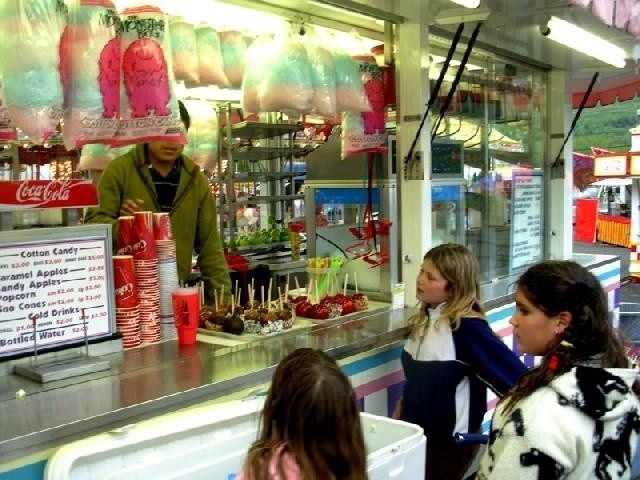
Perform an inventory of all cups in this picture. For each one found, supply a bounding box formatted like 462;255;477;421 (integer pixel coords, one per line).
112;210;202;352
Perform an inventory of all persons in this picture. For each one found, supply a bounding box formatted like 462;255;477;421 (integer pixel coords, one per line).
473;259;639;479
81;99;231;307
391;243;527;479
234;347;369;480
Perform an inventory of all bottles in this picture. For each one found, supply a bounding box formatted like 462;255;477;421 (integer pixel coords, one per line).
305;266;339;307
429;63;530;125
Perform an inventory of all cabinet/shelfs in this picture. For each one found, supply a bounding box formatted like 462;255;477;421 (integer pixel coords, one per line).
215;102;309;263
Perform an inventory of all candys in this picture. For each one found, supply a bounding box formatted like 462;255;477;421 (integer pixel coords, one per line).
201;272;368;335
